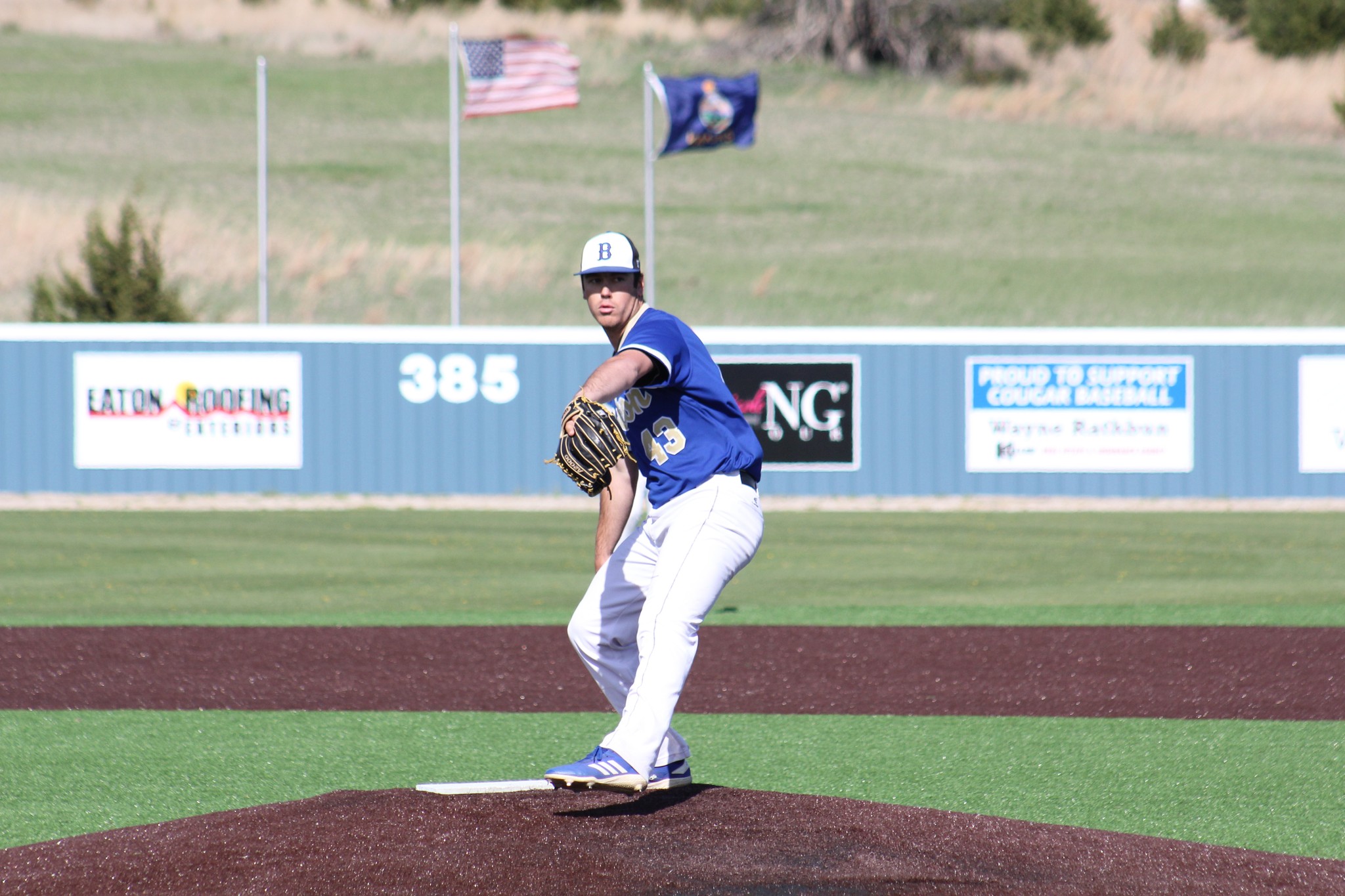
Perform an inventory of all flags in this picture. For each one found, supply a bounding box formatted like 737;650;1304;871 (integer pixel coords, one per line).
647;73;761;160
457;34;580;118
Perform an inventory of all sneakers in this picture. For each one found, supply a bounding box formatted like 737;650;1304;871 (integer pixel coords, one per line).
646;758;692;790
544;746;648;793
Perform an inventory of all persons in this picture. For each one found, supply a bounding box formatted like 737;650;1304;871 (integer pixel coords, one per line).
545;231;765;797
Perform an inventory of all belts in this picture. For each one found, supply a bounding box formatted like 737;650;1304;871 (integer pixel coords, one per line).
740;470;756;492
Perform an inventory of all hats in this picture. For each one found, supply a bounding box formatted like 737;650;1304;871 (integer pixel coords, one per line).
573;232;640;276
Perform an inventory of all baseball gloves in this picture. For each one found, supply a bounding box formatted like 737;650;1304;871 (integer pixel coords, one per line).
554;397;630;498
